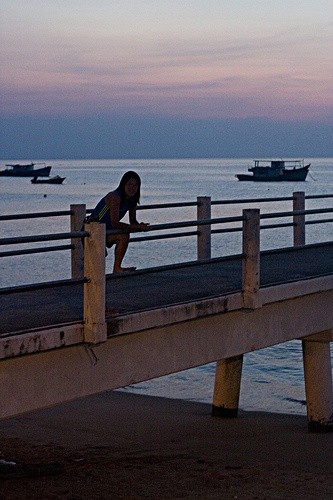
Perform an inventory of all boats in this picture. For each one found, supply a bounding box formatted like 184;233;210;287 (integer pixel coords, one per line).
31;176;65;184
0;162;52;177
235;159;318;181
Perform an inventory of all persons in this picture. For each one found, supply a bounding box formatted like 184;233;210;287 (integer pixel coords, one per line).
80;170;151;317
